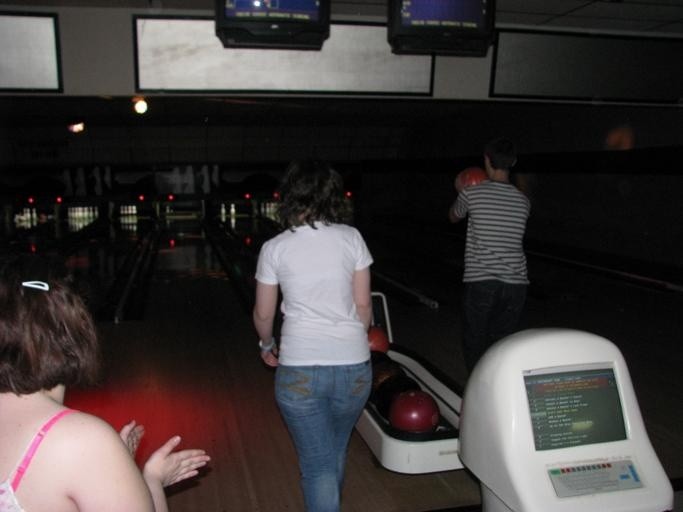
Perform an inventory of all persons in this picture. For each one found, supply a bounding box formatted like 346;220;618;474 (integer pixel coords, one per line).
0;276;213;510
251;160;376;512
443;134;532;376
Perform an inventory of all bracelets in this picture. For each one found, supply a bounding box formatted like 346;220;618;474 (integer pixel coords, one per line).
256;336;275;353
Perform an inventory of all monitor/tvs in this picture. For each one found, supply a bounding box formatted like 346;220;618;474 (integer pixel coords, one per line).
214;1;331;52
523;361;628;451
386;0;498;58
